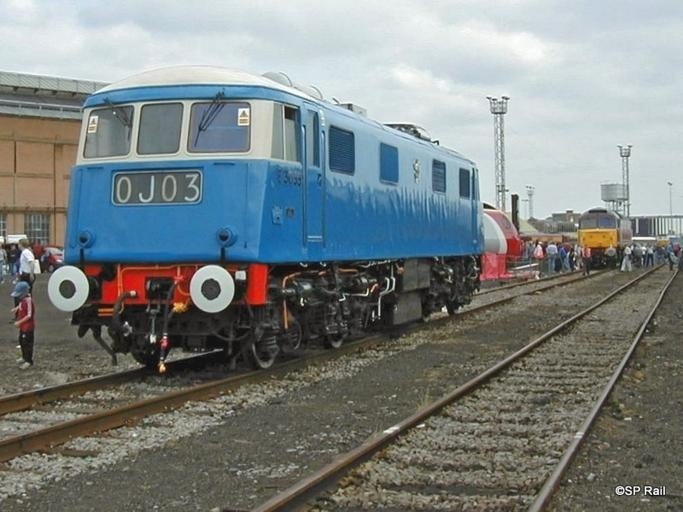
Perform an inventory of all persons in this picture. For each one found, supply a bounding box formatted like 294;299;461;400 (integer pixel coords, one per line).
521;237;681;277
0;237;59;369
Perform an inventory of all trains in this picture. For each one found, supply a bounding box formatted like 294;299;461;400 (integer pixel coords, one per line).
572;205;635;271
42;60;487;376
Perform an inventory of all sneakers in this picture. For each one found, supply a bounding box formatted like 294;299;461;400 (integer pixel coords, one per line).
19;362;30;370
16;357;25;363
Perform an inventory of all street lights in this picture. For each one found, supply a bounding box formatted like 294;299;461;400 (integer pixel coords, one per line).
523;184;535;219
484;94;511;214
615;143;633;219
667;181;672;217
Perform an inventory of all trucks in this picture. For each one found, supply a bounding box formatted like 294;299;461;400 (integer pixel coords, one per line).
33;245;64;274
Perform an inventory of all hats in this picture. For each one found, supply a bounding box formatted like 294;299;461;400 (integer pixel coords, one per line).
10;281;29;298
